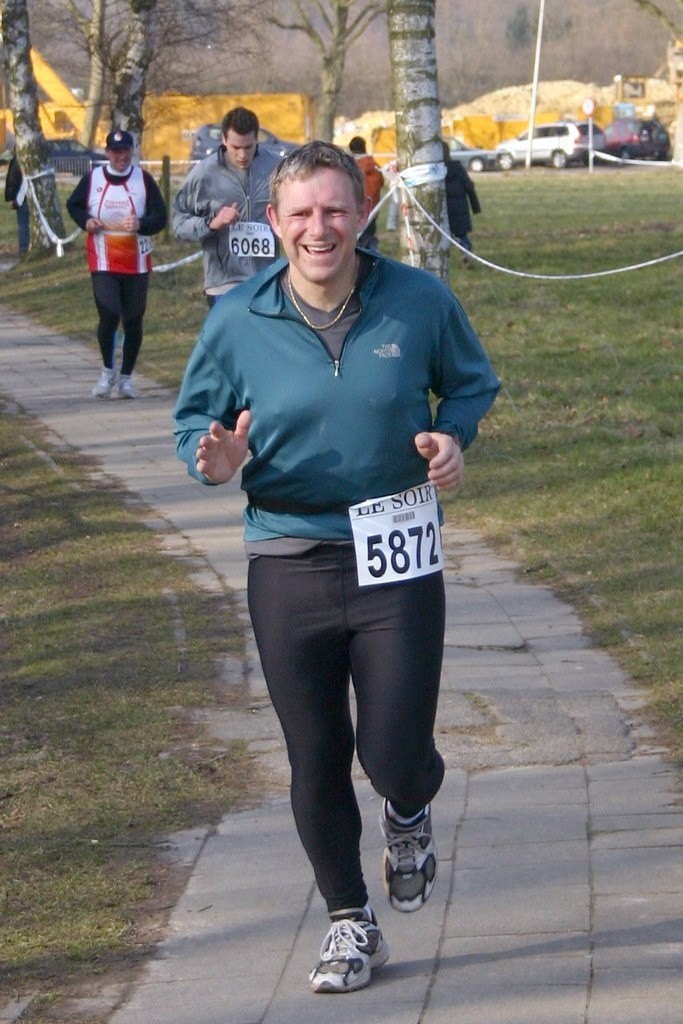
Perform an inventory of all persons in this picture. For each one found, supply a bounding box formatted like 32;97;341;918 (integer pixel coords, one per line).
172;140;501;993
173;106;284;310
66;130;168;399
5;146;30;256
346;136;384;247
442;141;481;269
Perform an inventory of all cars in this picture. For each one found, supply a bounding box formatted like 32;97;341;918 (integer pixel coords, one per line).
448;137;495;173
46;139;109;169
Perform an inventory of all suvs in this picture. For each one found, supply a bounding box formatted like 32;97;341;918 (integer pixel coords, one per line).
191;121;303;166
494;119;607;173
604;114;672;162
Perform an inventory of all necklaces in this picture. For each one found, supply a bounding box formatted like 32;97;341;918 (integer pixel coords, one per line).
288;273;356;329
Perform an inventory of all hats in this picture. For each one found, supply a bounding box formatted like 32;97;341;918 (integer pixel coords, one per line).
349;138;366;151
106;130;134;149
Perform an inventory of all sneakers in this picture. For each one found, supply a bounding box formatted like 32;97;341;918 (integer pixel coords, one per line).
309;907;389;991
117;374;137;398
380;798;437;910
93;366;115;398
